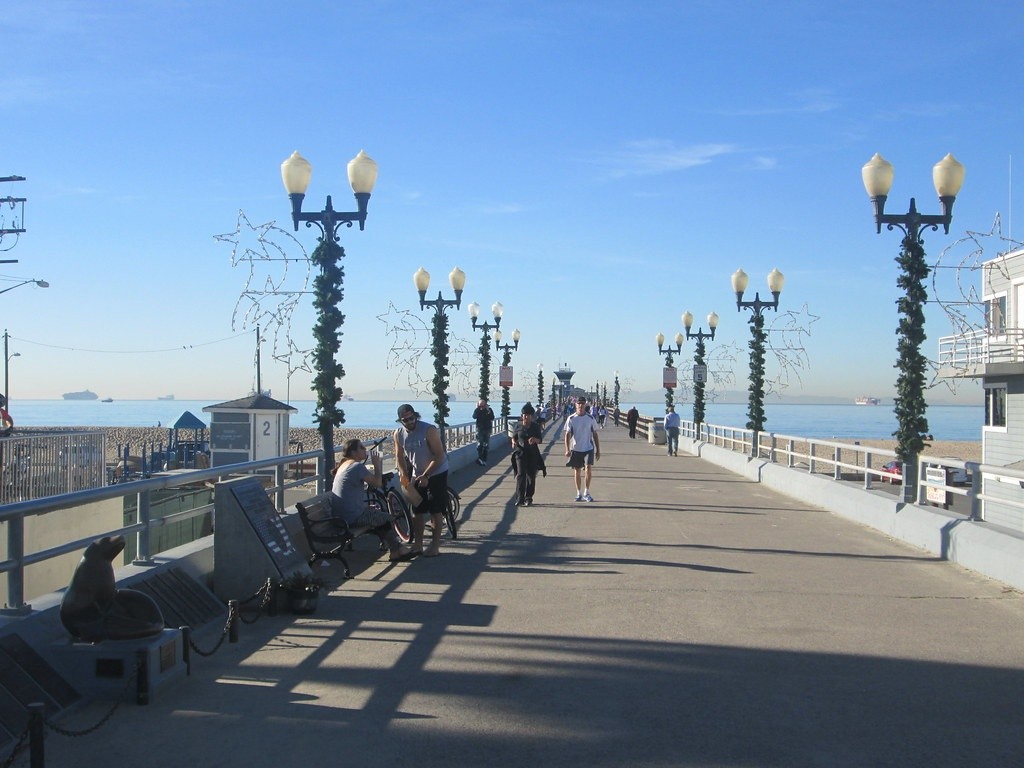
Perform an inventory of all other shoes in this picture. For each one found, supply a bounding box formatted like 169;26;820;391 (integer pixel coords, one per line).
668;451;677;457
514;500;532;506
573;494;593;502
477;459;486;466
409;544;438;557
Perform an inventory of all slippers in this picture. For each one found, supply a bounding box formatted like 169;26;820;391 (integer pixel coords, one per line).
390;549;422;562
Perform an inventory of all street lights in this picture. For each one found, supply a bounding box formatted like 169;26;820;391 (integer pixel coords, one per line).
551;376;556;407
656;332;684;414
861;151;964;457
603;381;607;406
682;310;720;423
413;267;465;428
586;379;603;407
560;381;566;404
281;148;377;493
613;369;621;405
493;328;520;416
731;268;784;431
537;361;544;406
468;301;504;408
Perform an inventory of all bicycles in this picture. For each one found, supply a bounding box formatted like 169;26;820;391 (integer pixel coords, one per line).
399;456;461;540
362;436;414;544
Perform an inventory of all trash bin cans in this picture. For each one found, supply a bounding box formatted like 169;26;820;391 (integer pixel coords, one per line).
649;423;667;445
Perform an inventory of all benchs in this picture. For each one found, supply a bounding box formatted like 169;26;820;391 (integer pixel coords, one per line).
295;489;405;580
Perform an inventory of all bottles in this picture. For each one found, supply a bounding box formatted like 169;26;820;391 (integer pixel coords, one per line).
367;451;384;458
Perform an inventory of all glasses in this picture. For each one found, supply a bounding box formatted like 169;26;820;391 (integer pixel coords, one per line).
402;415;415;423
360;445;365;450
577;403;585;405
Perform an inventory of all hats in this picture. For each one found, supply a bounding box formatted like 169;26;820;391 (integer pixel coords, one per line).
398;404;414;418
579;397;586;402
521;403;535;414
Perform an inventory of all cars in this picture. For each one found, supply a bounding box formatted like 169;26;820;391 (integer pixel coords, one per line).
925;457;968;486
880;462;903;484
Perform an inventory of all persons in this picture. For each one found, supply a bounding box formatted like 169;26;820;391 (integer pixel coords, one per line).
627;407;639;439
511;402;542;507
664;406;680;456
330;439;420;562
564;397;600;502
534;396;619;430
393;404;449;557
473;400;495;467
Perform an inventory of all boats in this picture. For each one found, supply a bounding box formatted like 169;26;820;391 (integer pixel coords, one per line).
101;397;113;402
856;396;880;407
158;393;174;400
62;389;98;401
